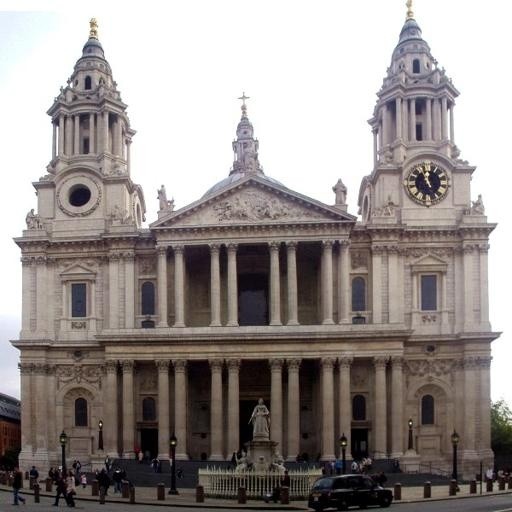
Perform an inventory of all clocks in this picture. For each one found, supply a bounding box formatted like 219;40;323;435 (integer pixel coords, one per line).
403;161;450;207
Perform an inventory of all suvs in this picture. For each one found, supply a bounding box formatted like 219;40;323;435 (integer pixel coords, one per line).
307;474;394;512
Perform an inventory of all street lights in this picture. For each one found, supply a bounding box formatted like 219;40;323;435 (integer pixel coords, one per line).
167;433;181;495
338;432;347;474
408;416;415;449
97;419;104;450
59;429;69;480
450;424;460;492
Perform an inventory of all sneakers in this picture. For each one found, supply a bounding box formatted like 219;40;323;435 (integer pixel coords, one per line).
12;503;19;505
52;504;58;506
22;499;26;504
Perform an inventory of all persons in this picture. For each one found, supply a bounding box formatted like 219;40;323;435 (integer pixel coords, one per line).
280;470;290;492
11;466;26;505
176;465;185;481
320;454;388;488
104;455;112;474
271;449;286;470
48;459;134;507
25;208;39;229
381;194;400;217
247;396;271;441
30;465;42;489
234;447;248;472
110;203;122;221
156;184;168;211
332;177;347;204
507;468;512;482
469;193;485;214
253;454;269;496
485;465;493;492
120;444;161;473
497;465;506;482
